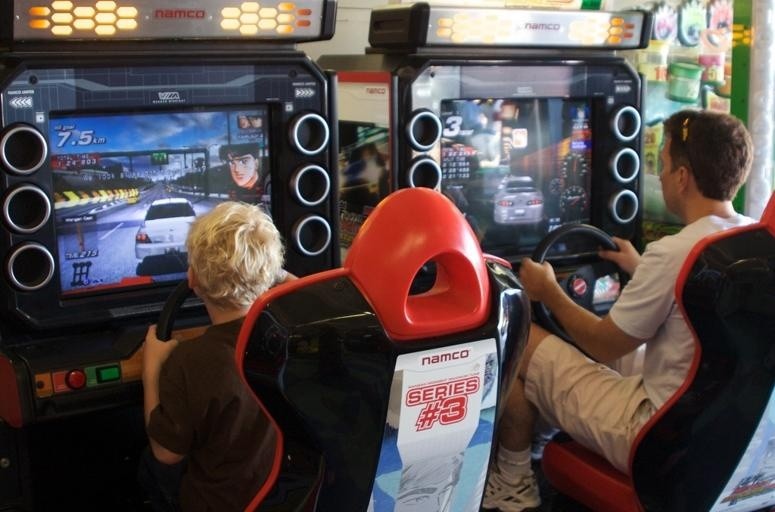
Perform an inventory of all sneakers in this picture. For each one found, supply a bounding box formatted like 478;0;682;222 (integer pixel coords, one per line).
530;434;555;459
482;463;542;512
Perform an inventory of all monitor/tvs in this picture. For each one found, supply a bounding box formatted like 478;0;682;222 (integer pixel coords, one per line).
49;105;272;296
441;98;593;256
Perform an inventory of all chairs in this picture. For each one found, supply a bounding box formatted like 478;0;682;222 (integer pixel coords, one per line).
236;187;530;512
542;189;775;512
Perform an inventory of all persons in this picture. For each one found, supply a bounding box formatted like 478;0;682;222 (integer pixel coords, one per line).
480;108;761;512
140;199;286;512
